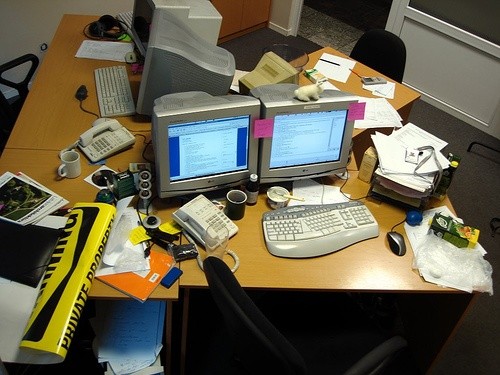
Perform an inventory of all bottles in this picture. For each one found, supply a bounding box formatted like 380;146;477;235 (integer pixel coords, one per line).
245;174;259;206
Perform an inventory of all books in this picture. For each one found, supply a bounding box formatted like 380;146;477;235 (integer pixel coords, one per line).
93;248;176;304
0;170;63;226
8;172;70;227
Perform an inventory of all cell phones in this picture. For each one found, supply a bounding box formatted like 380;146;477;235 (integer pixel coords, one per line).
362;76;387;85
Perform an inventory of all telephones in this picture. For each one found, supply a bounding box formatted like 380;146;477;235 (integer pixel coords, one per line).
171;193;240;273
58;119;137;164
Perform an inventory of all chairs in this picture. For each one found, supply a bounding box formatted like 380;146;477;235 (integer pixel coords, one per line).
350;29;407;83
0;54;40;154
204;257;406;374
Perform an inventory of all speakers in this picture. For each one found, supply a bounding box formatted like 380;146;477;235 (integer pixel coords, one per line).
136;162;153;215
335;139;353;180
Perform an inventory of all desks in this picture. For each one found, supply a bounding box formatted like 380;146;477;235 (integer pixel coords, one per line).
0;15;179;375
177;152;491;373
286;47;423;139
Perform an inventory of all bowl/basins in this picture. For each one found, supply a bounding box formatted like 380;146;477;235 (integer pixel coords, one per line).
267;186;290;210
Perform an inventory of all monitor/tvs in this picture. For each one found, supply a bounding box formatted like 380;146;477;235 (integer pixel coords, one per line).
238;49;301;96
152;90;262;200
248;82;360;184
130;0;223;56
134;8;236;115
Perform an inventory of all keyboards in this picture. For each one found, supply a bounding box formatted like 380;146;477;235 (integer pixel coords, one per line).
117;11;133;36
262;200;381;258
94;65;135;118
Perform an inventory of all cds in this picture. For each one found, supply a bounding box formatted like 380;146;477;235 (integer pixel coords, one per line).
93;170;117;187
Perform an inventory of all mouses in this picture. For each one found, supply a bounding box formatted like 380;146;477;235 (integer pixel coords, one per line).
75;84;88;102
92;116;115;126
386;230;408;257
125;52;137;64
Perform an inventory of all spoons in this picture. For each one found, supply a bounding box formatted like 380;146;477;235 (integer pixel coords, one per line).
275;190;305;201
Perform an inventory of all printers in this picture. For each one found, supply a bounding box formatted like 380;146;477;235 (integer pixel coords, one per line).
358;127;457;213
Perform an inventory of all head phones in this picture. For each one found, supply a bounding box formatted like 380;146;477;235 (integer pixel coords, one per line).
89;14;125;38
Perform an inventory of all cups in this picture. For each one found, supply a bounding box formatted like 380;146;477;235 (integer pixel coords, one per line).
58;151;81;179
262;44;309;85
226;190;248;220
206;224;229;260
